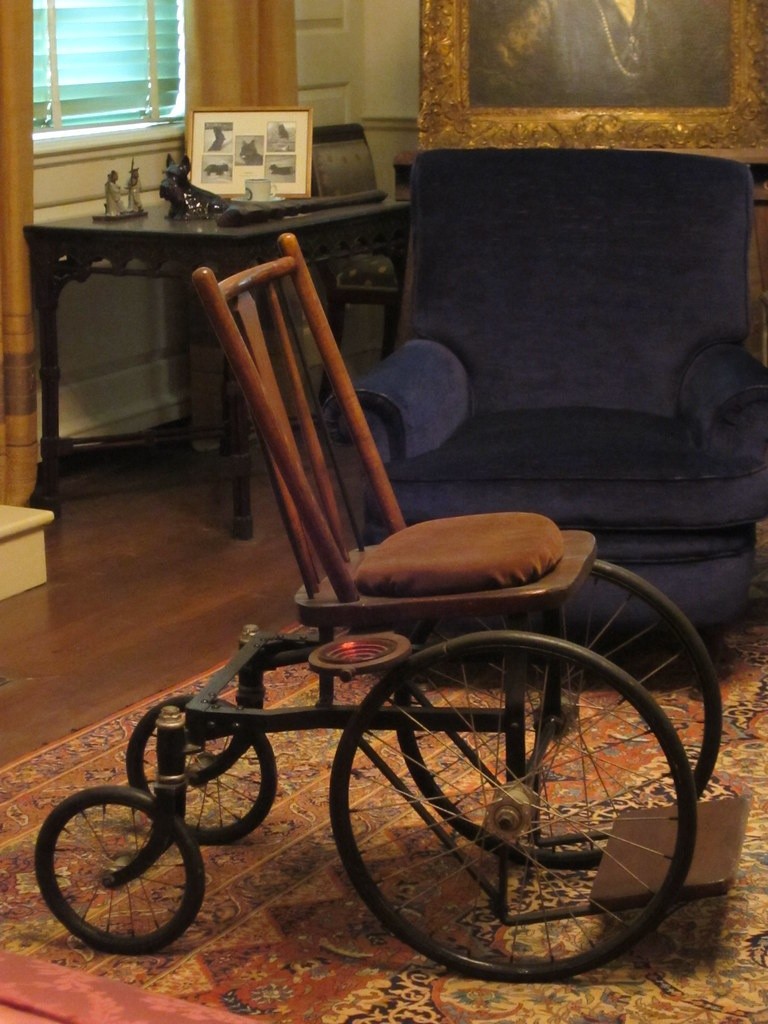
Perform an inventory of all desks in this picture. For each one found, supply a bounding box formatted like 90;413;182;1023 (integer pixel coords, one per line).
23;200;408;541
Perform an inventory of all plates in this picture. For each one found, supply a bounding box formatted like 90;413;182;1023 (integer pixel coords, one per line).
231;197;285;201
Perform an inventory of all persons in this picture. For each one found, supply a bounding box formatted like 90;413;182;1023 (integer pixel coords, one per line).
105;171;127;216
124;167;144;212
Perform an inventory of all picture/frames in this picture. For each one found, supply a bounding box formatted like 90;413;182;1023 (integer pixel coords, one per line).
417;0;768;165
185;105;312;200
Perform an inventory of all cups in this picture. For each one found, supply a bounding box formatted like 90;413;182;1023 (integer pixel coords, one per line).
245;179;277;200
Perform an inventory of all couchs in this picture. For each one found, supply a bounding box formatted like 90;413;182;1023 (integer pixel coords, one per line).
317;143;768;670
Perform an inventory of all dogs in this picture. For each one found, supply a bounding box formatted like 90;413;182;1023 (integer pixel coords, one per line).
159;155;228;221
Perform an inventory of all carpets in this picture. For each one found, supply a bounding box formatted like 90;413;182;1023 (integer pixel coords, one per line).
0;624;768;1024
